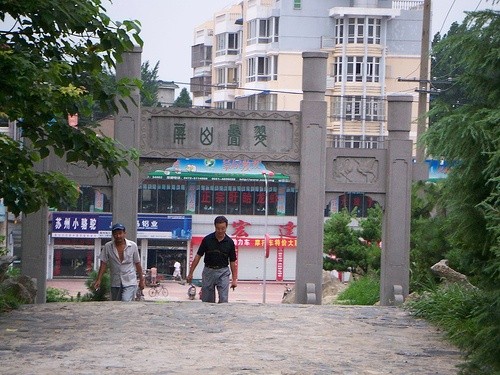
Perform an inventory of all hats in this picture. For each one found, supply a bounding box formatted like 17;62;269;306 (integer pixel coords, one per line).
112;223;125;230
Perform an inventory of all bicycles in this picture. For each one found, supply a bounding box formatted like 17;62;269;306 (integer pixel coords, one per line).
149;285;169;297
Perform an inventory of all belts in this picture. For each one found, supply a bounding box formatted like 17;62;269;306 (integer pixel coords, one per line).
205;265;228;269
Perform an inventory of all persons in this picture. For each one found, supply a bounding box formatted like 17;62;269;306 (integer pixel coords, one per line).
173;260;182;282
93;223;145;302
186;214;238;303
150;265;157;284
